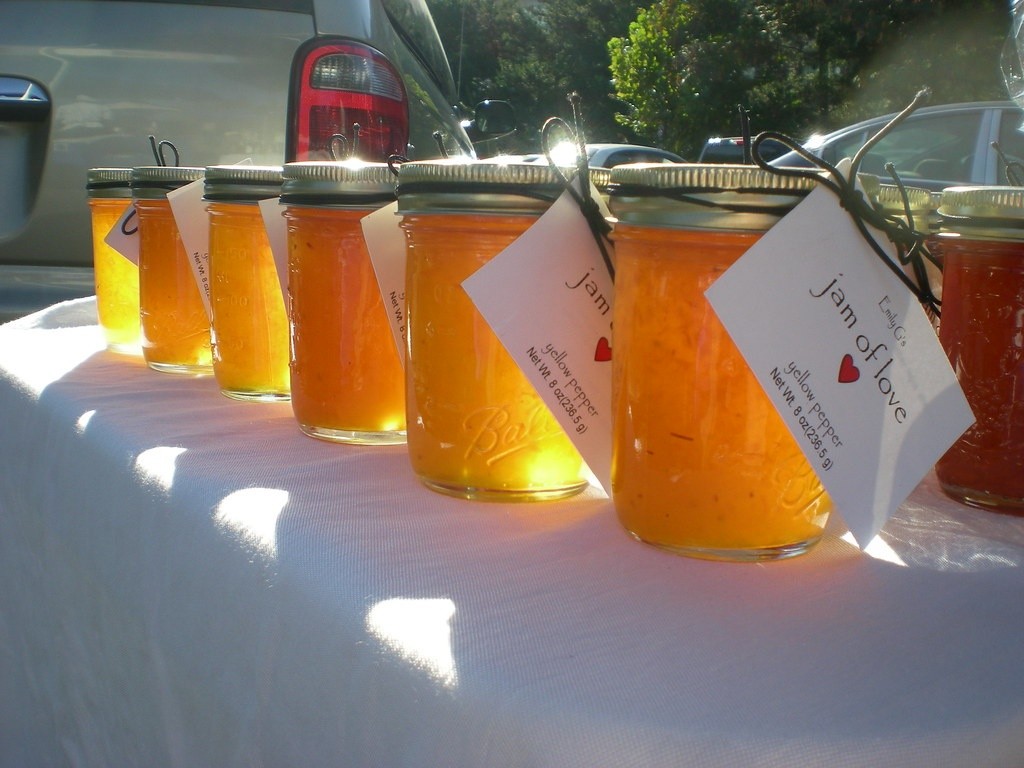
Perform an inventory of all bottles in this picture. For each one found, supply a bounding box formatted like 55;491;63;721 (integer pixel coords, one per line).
130;165;216;376
875;180;1024;520
84;167;144;358
392;160;612;503
606;159;867;562
204;163;295;404
281;160;420;446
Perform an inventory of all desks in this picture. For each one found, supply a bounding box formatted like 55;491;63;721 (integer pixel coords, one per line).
0;293;1024;767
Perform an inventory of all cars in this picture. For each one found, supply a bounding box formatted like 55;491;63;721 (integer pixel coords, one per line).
0;1;521;327
755;99;1024;201
695;136;791;169
531;141;690;174
